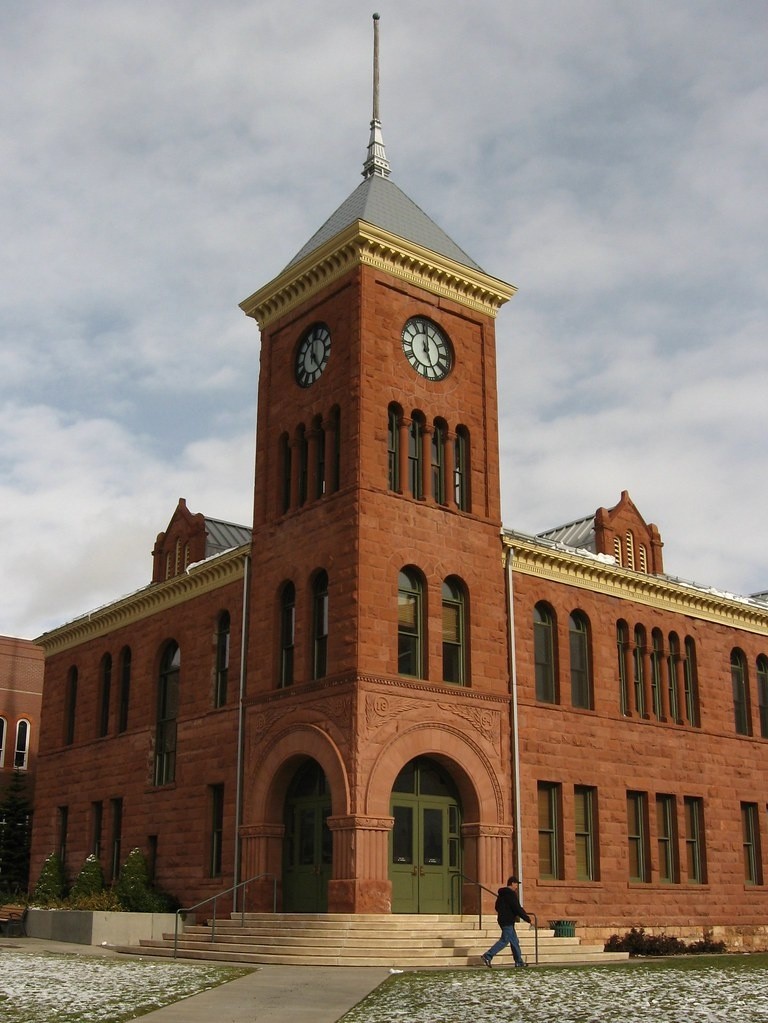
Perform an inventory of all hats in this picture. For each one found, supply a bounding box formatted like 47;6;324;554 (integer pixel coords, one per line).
509;876;522;884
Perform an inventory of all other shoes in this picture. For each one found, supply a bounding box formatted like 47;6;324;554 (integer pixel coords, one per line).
515;963;529;967
481;955;492;969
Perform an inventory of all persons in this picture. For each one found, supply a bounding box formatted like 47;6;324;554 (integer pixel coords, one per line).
480;876;535;967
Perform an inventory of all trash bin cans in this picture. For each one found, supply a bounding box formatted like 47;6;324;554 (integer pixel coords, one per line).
547;918;577;939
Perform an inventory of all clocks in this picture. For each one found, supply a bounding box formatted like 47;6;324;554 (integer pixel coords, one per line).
295;330;330;386
401;318;451;382
